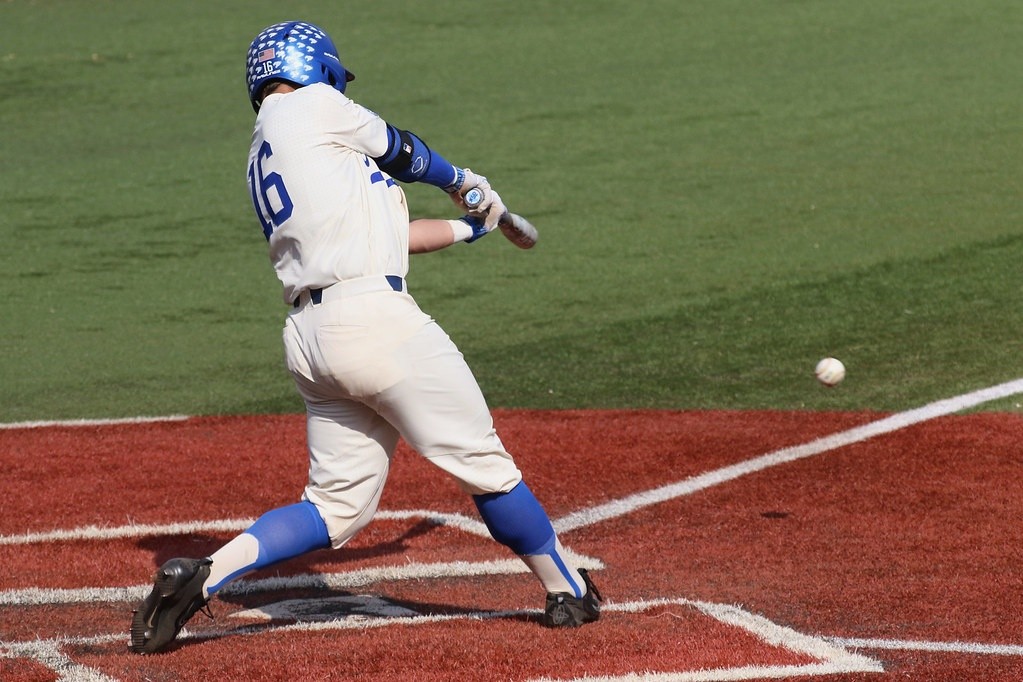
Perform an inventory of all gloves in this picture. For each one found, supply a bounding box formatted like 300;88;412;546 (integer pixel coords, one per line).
442;165;493;217
459;189;508;243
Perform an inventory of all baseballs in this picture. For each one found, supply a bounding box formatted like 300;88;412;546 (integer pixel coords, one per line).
815;357;846;386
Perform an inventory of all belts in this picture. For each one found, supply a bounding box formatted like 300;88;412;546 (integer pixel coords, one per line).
294;275;402;307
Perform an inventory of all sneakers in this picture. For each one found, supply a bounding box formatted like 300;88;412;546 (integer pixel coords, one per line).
126;556;214;653
546;568;604;627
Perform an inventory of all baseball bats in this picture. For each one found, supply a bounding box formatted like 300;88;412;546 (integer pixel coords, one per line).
462;187;540;252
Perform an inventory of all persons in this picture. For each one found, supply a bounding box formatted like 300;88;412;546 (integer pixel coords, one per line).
130;19;606;654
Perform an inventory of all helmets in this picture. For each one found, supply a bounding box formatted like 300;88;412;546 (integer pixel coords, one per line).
245;20;355;115
